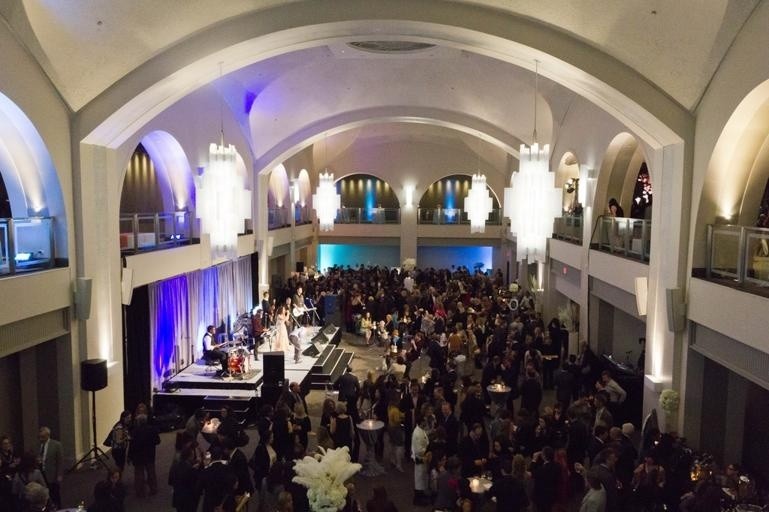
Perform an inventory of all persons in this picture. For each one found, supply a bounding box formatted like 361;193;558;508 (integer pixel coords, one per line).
1;262;769;511
756;214;769;258
608;198;626;253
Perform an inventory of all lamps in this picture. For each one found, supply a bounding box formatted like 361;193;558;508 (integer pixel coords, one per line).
633;276;648;316
464;134;493;234
503;60;563;265
74;277;92;321
120;266;134;306
311;133;341;232
266;235;276;257
564;175;580;204
195;61;252;257
665;286;688;333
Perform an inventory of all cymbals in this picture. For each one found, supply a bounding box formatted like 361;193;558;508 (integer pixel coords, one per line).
230;341;242;344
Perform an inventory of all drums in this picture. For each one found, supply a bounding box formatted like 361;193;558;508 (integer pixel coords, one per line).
228;347;249;373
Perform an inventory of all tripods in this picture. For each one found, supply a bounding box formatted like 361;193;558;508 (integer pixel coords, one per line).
65;390;113;476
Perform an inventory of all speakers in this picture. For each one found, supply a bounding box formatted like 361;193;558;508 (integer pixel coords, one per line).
302;341;323;358
319;322;337;334
296;262;304;273
325;295;341;325
81;359;107;391
264;352;284;384
311;331;329;344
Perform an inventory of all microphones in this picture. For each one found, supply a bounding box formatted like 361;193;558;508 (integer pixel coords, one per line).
306;297;312;300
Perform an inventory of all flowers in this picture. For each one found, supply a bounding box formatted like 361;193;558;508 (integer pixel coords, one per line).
659;389;680;410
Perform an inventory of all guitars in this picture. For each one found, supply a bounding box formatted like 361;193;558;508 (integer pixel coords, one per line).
293;307;317;317
268;299;276;323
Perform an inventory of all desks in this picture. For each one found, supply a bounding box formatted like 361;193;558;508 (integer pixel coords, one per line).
355;419;387;477
486;383;512;429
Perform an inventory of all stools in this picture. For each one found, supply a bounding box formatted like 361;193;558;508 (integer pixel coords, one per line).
204;360;220;377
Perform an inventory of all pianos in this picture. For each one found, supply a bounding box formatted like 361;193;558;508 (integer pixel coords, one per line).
260;326;276;338
